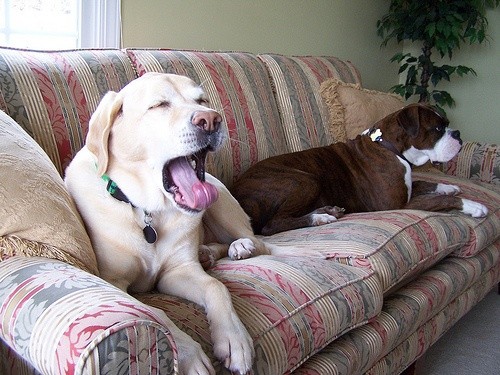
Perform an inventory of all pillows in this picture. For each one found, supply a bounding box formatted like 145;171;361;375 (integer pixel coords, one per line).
0;110;99;276
320;74;407;148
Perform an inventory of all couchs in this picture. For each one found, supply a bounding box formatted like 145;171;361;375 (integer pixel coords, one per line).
0;46;500;374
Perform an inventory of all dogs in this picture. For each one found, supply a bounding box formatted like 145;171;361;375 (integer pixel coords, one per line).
63;72;326;375
229;102;488;236
